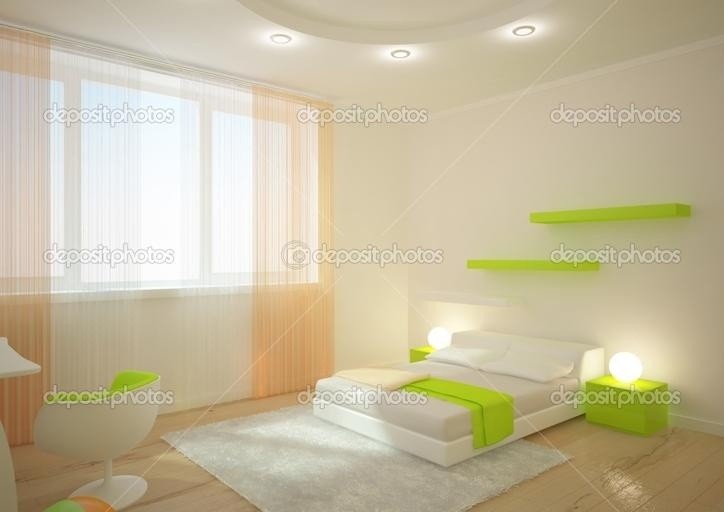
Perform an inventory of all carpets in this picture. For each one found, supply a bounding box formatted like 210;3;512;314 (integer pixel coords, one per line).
160;400;572;511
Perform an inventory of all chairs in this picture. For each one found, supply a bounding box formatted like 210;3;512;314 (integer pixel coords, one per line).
32;370;162;511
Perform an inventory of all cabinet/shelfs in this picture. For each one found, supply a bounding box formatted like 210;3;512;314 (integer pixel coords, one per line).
465;202;691;275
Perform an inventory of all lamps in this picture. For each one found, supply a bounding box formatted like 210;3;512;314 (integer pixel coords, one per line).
427;327;452;350
608;351;645;383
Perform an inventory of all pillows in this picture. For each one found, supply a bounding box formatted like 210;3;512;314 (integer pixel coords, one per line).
425;344;577;384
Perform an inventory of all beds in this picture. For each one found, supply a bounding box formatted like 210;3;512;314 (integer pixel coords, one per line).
312;330;606;468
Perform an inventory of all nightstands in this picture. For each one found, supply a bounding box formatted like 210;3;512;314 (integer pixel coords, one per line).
584;375;668;436
409;346;431;363
